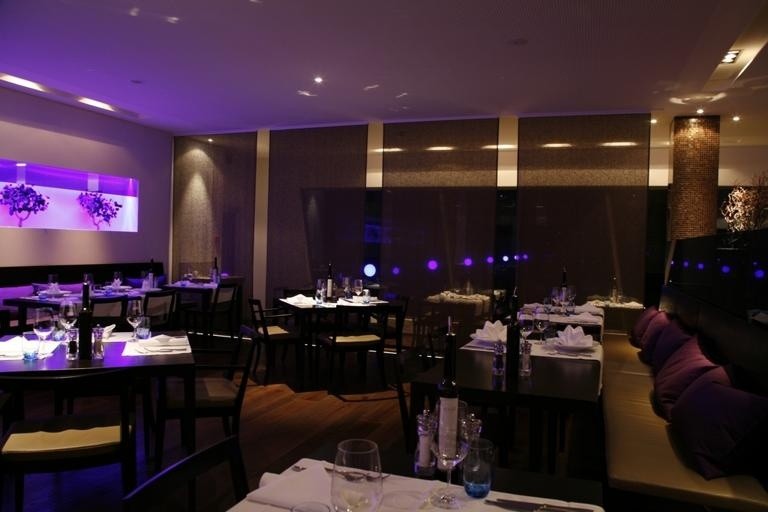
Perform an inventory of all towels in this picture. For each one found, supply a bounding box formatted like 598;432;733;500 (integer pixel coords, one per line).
469;318;508;343
246;461;334;512
551;323;594;351
137;335;187;347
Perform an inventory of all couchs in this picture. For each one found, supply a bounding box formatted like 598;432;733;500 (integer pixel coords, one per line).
603;292;768;509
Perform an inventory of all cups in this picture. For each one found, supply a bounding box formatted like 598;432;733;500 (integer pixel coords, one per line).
331;440;384;511
59;302;77;345
134;317;152;342
517;308;534;351
127;300;143;342
430;399;472;510
533;307;550;345
544;322;556;352
461;440;494;500
21;332;40;361
33;308;55;358
51;318;67;341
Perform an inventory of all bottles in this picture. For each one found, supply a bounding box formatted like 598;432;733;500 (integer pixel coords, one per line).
463;417;480;455
520;340;533;375
560;265;567;302
439;332;458;460
611;272;618;301
327;264;332;301
333;283;337;298
78;282;92;360
419;414;436;466
212;257;218;284
150;259;156;288
467;271;472;295
491;340;504;375
65;330;78;360
506;295;519;377
94;324;105;359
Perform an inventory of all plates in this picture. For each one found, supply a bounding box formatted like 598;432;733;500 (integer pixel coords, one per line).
470;334;506;346
546;338;600;351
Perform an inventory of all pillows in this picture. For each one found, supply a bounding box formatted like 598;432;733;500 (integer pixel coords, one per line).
638;310;670;350
653;335;720;421
670;364;735;426
639;319;691;375
688;381;767;480
627;305;659;342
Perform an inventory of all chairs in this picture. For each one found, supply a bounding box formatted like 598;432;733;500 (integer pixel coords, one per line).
152;324;261;498
143;260;175;316
318;305;382;389
213;256;247;306
1;367;139;512
247;297;292;384
122;433;250;512
375;293;410;356
